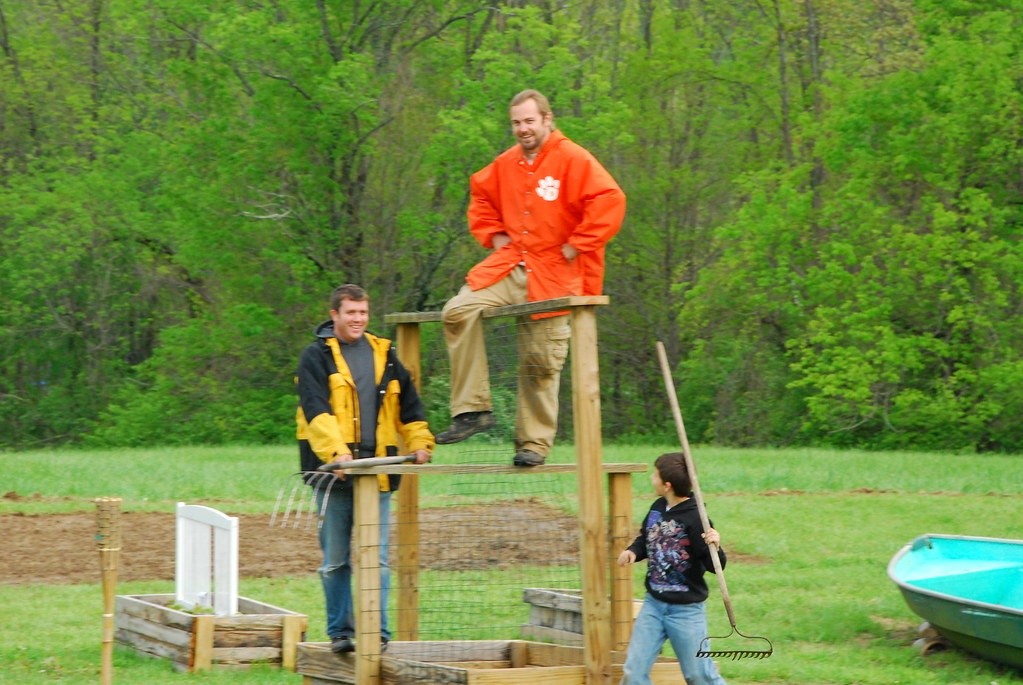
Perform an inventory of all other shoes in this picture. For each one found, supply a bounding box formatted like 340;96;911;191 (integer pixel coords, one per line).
433;409;496;445
513;449;544;465
330;636;355;653
380;639;388;654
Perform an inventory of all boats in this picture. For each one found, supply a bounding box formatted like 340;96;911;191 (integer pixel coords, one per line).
886;531;1023;673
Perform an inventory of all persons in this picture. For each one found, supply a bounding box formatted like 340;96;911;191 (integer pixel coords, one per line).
294;284;436;653
433;89;626;467
617;452;727;685
